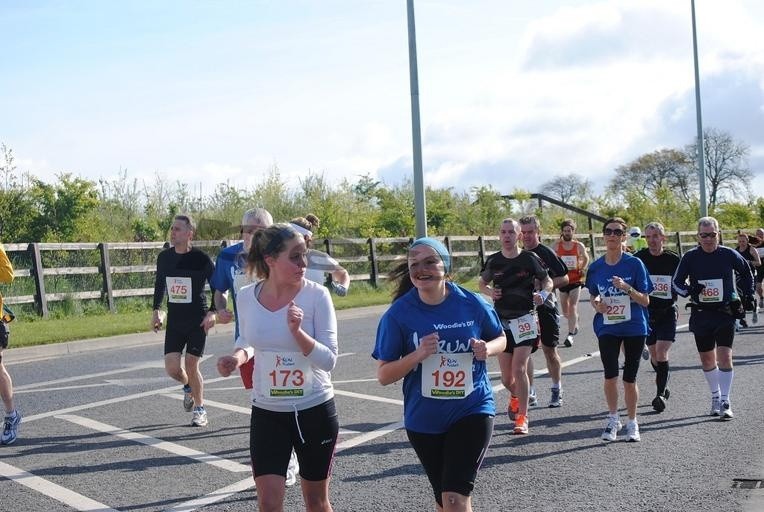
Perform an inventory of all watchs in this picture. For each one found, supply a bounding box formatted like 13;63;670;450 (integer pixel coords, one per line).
627;287;633;296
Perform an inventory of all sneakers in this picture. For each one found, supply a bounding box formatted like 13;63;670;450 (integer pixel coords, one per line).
512;415;529;434
1;411;22;446
625;423;641;442
563;335;576;347
752;312;758;323
508;397;520;420
191;407;208;427
709;403;722;415
548;397;564;407
665;388;670;399
183;385;194;411
600;421;622;441
527;395;538;410
652;395;667;411
720;401;732;420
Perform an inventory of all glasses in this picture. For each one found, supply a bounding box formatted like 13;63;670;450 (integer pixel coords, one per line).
603;228;625;236
698;232;719;238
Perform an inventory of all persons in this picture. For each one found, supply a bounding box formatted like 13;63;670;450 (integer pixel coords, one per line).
633;222;681;412
371;237;507;512
289;213;350;297
153;216;218;427
479;219;553;433
734;233;761;327
671;217;755;419
210;209;299;487
554;220;589;346
218;224;339;512
585;217;654;443
521;214;569;408
625;226;648;256
753;228;764;309
0;245;22;446
619;242;649;369
